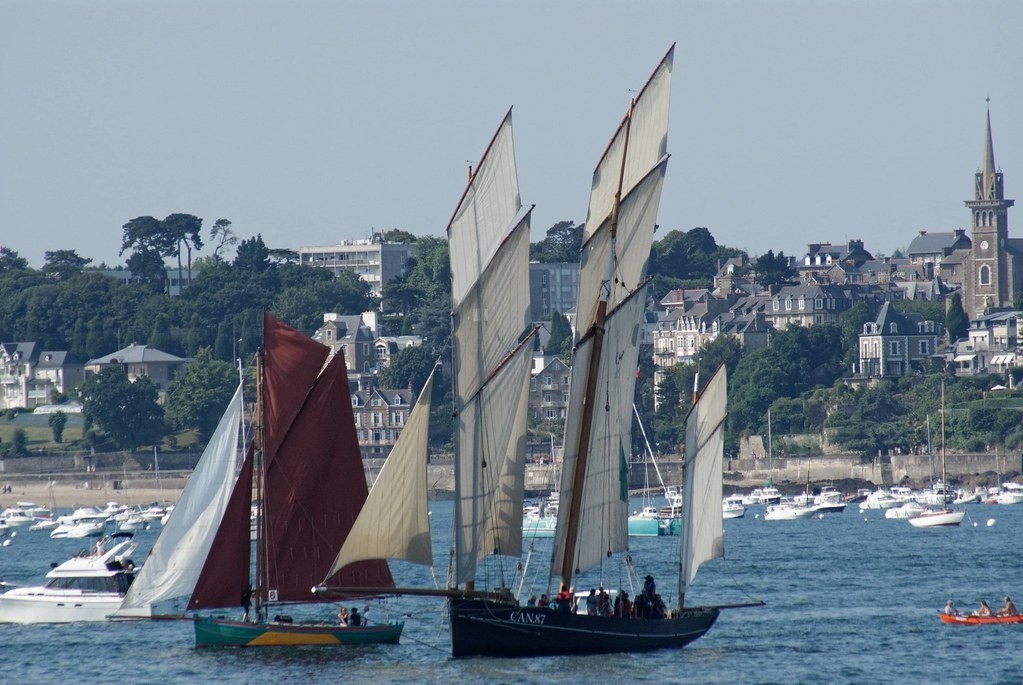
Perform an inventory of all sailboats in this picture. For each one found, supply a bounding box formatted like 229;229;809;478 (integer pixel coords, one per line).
102;309;411;649
310;43;769;661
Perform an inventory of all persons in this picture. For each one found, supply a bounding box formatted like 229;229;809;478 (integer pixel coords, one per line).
363;606;374;626
997;597;1017;616
338;608;348;626
979;601;990;616
93;535;108;556
2;485;11;494
350;608;361;626
589;575;666;619
527;585;576;610
945;600;958;616
114;557;135;592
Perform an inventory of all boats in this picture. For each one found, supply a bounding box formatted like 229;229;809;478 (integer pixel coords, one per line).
0;529;173;624
519;375;1023;540
936;609;1023;627
0;448;259;542
